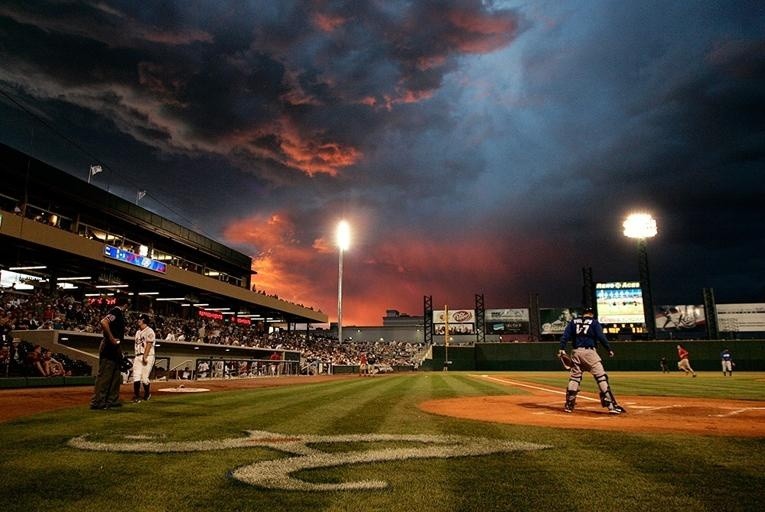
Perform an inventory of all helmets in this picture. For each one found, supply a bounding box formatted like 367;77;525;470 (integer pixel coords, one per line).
136;314;149;322
582;306;594;314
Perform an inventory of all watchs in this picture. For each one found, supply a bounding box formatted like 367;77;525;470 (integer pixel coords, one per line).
443;360;447;372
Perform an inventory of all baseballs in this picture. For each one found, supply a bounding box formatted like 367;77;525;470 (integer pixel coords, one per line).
116;340;120;344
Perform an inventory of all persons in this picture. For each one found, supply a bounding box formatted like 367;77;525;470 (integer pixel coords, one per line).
720;348;732;376
541;311;577;334
660;356;670;373
679;312;698;326
661;308;678;329
89;291;124;409
121;349;133;384
434;325;545;346
311;334;425;377
1;202;323;379
558;304;626;415
676;344;696;378
611;299;639;310
131;312;157;404
440;362;442;373
491;310;524;320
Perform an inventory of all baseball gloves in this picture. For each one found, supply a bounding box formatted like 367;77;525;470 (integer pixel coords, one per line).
559;354;573;371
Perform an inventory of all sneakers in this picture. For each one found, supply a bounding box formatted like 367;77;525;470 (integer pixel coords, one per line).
89;404;123;411
143;392;152;401
563;405;573;413
129;395;142;405
608;406;626;414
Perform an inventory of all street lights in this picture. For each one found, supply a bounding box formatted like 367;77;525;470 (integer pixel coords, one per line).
622;212;658;336
335;218;351;344
